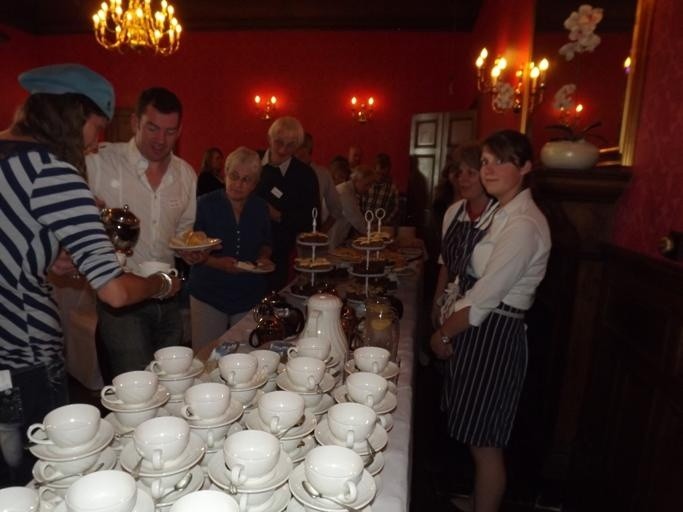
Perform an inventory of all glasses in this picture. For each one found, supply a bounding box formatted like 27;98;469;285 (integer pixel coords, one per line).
227;171;257;186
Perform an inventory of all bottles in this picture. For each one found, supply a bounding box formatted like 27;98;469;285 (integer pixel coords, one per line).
99;204;139;254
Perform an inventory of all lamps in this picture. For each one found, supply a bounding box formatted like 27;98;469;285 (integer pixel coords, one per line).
349;93;377;126
253;91;277;122
473;36;550;112
89;1;182;58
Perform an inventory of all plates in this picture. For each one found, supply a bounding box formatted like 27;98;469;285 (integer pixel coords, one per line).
328;240;425;277
170;240;219;251
288;285;308;299
369;232;393;244
344;296;363;304
296;239;328;247
238;266;275;275
293;264;333;273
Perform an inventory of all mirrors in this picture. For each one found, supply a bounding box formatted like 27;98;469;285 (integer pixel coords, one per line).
521;1;656;167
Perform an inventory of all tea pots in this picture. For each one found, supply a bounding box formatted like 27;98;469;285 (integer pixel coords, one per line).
293;290;348;371
249;288;302;348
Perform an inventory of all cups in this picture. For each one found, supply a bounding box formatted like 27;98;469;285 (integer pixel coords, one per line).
362;298;400;360
380;226;415;239
0;338;399;511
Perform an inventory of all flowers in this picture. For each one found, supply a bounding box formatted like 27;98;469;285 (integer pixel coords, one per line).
544;3;607;142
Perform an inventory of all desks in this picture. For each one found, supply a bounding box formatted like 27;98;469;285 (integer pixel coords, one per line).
21;225;428;511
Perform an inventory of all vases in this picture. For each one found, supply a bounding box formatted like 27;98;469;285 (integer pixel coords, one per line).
539;138;601;172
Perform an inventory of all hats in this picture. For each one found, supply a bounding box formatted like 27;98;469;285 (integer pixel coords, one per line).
17;62;116;123
